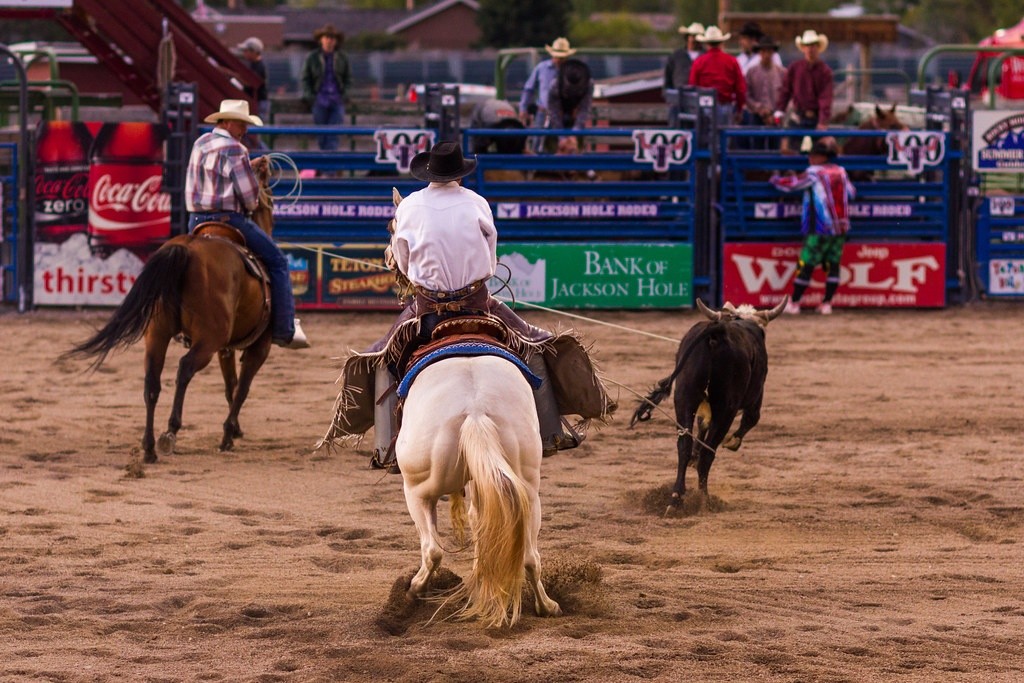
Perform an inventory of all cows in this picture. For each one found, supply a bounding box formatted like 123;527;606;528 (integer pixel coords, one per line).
628;296;787;517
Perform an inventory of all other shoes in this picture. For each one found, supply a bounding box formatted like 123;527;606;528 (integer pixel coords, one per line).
814;303;832;314
286;318;311;348
783;297;800;314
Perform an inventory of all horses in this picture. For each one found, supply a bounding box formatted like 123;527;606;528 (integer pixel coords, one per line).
383;186;566;628
743;101;910;185
58;155;277;465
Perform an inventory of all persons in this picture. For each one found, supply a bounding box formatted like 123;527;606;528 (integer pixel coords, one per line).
175;99;309;348
665;22;747;177
237;35;269;150
778;29;837;152
770;136;856;315
302;23;350;176
733;21;789;149
520;37;595;155
374;139;585;477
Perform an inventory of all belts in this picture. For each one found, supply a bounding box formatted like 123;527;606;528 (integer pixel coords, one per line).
197;210;235;215
421;281;482;298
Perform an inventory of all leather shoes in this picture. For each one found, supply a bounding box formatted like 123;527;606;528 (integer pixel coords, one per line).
544;432;586;456
371;459;402;474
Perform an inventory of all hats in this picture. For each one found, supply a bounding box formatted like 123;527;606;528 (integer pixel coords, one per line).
696;25;731;42
314;23;344;50
546;36;576;58
238;36;264;54
410;141;477;183
796;30;828;53
678;22;704;35
203;99;264;127
740;22;765;41
753;36;780;53
815;135;838;152
